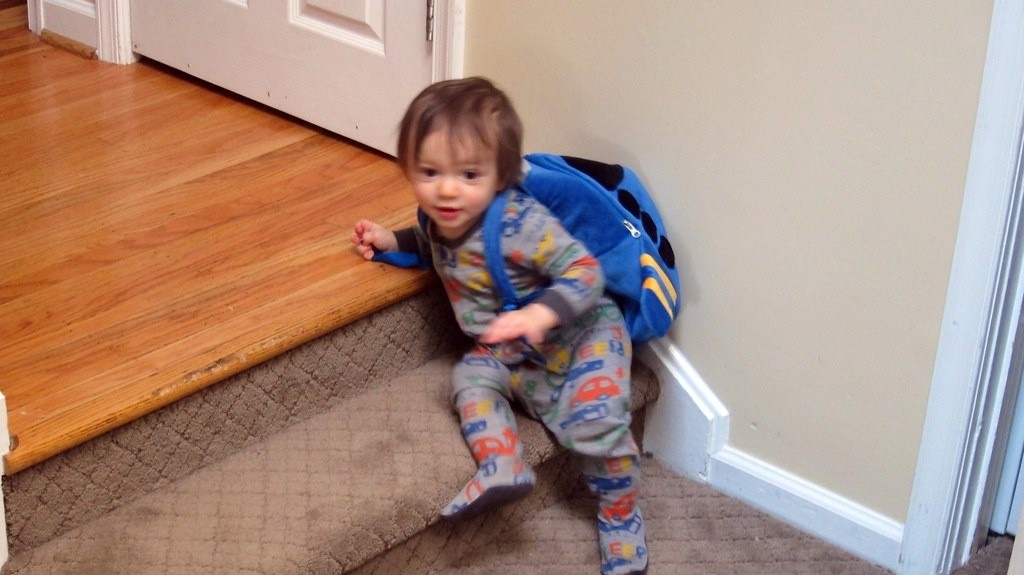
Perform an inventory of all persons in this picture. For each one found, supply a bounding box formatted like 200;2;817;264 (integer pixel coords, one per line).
352;76;647;575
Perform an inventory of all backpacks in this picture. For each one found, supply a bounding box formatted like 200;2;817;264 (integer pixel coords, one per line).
417;153;681;349
